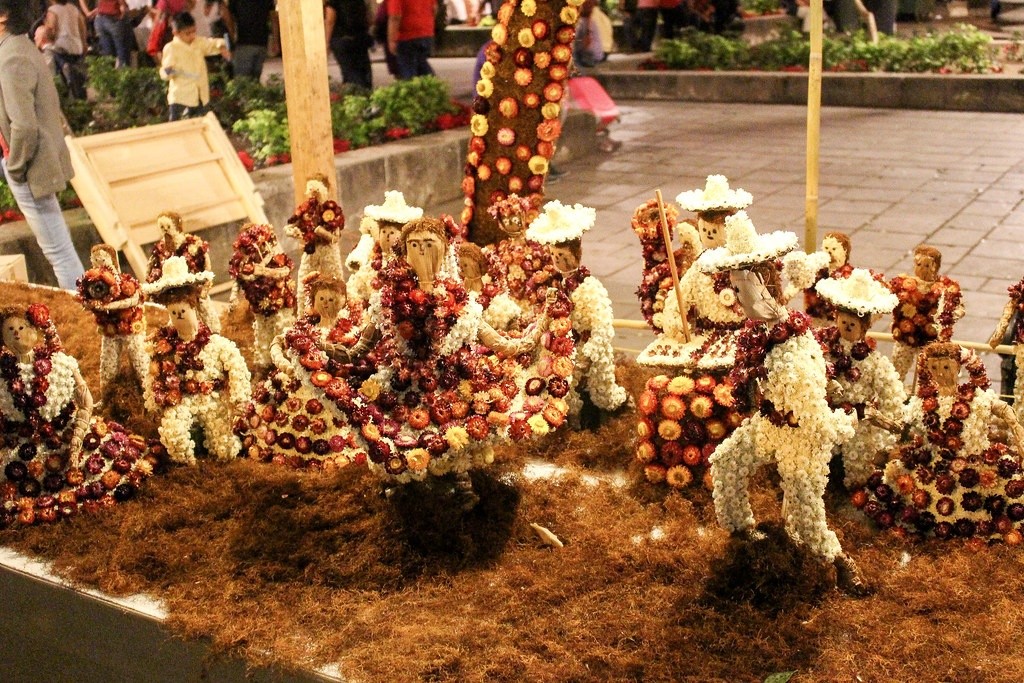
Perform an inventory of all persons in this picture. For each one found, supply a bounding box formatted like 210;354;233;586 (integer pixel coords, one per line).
696;211;869;597
348;190;421;327
454;241;522;331
29;0;275;121
664;178;748;342
868;343;1023;543
144;257;252;463
814;269;905;488
228;222;296;383
805;233;852;329
481;196;552;278
291;217;572;509
73;243;155;414
0;303;160;523
285;172;345;320
237;272;361;469
144;212;223;335
321;0;745;185
868;246;967;381
991;281;1024;404
0;0;85;290
524;200;636;430
